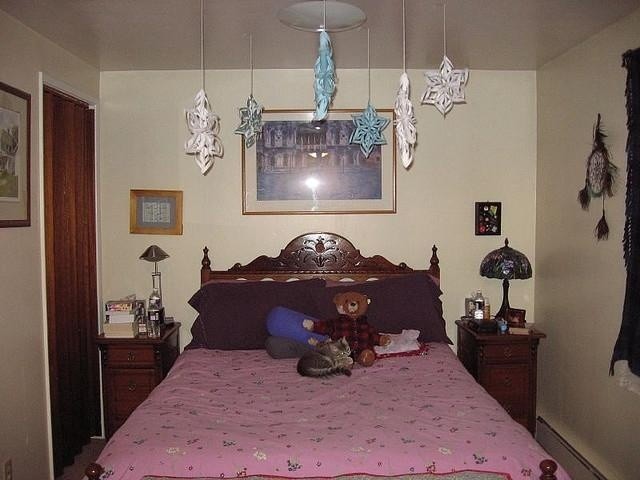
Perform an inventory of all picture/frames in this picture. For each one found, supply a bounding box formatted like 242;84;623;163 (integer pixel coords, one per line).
1;82;32;228
129;188;184;237
241;107;397;216
475;200;504;238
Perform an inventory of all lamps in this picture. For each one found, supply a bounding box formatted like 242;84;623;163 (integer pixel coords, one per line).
139;243;173;327
479;236;535;322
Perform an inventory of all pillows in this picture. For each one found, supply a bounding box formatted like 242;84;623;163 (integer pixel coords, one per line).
326;267;455;346
266;306;330;352
183;278;341;351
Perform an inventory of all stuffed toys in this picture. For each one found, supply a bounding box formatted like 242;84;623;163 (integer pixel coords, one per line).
302;291;392;367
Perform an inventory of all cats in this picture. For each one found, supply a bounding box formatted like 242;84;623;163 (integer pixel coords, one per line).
296;335;354;380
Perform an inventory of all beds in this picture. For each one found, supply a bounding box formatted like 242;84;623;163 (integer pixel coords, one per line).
84;229;570;480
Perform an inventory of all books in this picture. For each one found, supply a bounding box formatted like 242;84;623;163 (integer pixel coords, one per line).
103;299;142;340
508;321;537;336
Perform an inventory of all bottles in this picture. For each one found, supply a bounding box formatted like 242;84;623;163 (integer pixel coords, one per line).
484;299;490;320
149;287;161;305
147;300;160;337
473;290;484;319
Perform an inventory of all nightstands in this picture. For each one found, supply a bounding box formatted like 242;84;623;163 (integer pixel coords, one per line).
457;319;547;439
94;322;183;445
